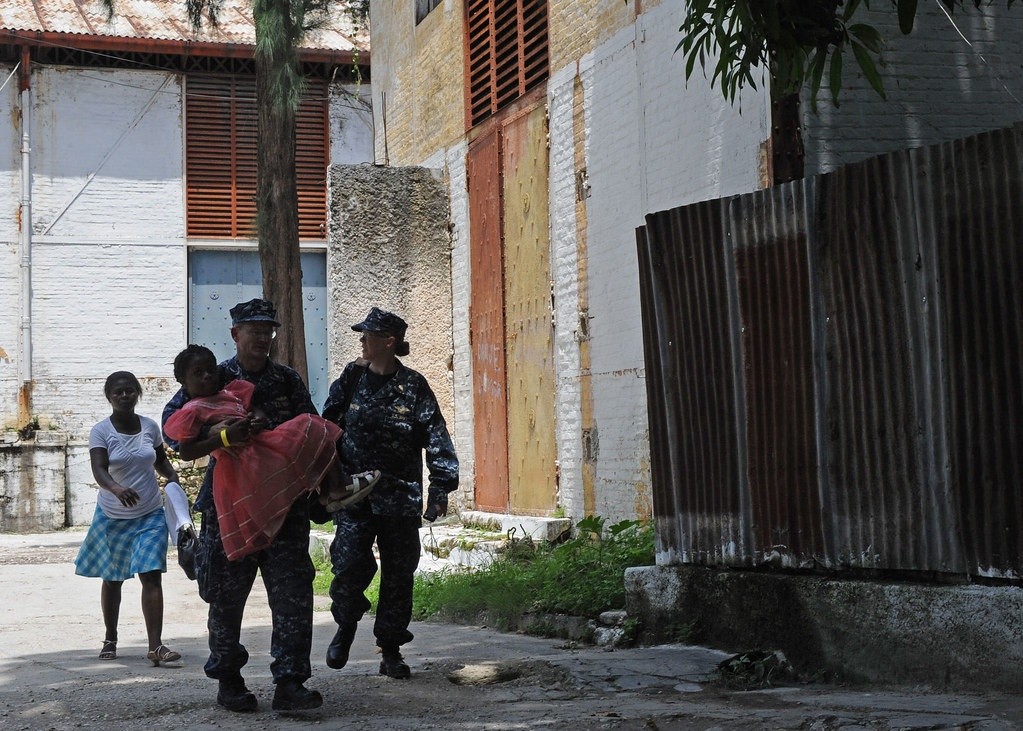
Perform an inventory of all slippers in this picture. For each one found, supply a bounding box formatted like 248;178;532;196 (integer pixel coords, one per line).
325;469;381;512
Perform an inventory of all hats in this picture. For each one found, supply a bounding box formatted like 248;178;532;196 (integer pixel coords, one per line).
229;299;282;328
351;307;409;339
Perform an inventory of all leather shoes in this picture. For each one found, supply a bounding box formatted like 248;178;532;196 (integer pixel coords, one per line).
270;679;323;712
326;619;358;669
379;647;411;679
217;675;259;712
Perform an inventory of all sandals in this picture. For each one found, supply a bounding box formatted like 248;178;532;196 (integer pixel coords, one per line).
148;644;181;665
98;640;118;660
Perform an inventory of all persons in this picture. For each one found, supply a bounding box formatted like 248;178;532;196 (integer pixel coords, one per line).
321;307;459;679
161;300;323;713
73;371;181;665
166;345;382;513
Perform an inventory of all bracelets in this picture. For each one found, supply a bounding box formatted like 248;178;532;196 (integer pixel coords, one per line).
221;429;230;447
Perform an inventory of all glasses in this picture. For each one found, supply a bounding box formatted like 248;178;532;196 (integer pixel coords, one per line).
250;328;276;340
